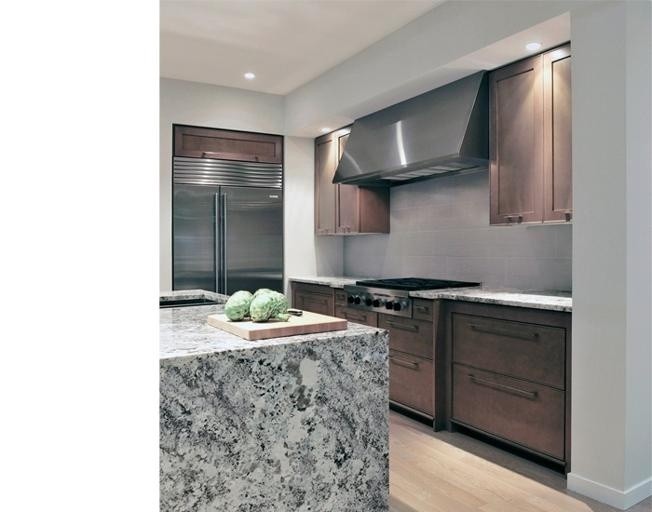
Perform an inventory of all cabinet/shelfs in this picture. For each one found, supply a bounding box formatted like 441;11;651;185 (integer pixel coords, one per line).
292;289;333;316
334;306;376;325
173;126;282;164
450;310;566;461
487;43;571;227
376;313;432;417
313;124;389;236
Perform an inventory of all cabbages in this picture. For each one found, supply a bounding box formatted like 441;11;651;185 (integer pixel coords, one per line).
225;288;288;323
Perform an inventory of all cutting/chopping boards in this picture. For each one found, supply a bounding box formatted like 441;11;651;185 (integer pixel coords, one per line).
207;308;347;341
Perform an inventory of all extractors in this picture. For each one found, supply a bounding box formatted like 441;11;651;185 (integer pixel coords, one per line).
332;70;490;189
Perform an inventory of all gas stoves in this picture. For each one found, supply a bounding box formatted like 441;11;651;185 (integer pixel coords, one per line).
342;276;480;319
